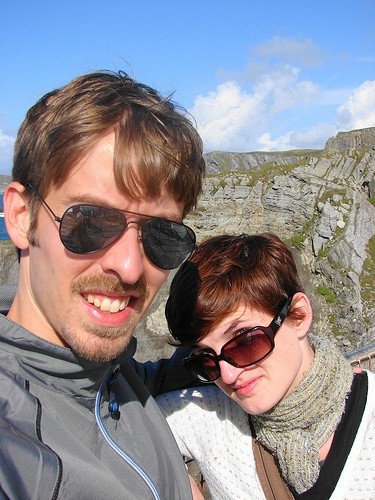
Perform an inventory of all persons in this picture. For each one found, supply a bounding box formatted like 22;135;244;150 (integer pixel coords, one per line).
0;69;213;500
154;233;375;500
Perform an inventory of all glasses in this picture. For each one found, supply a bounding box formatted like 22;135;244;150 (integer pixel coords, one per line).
24;182;199;270
180;294;293;383
